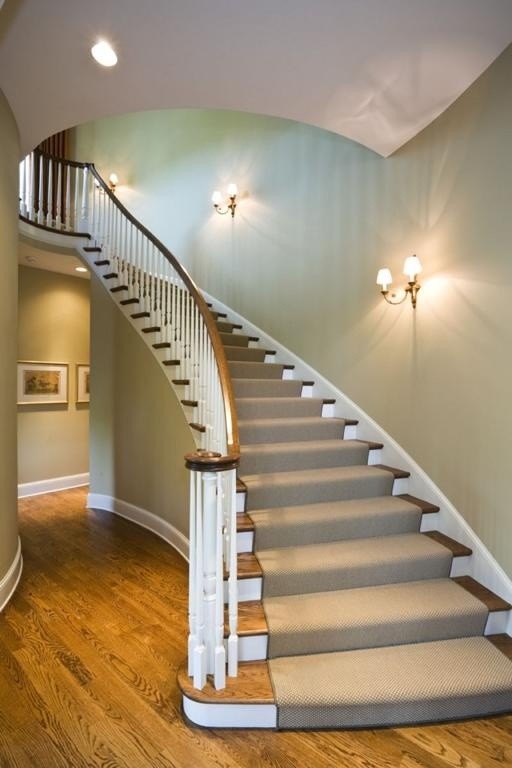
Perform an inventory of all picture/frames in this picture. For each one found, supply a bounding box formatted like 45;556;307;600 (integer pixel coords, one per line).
75;362;90;404
15;360;70;406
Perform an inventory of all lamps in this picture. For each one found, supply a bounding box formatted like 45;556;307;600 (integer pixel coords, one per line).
90;173;119;199
375;249;424;307
210;183;239;219
